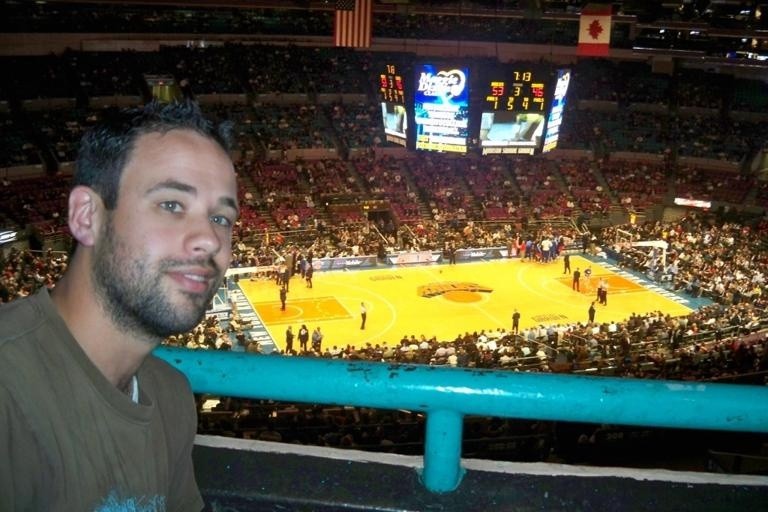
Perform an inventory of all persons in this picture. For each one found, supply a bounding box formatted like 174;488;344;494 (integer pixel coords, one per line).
1;0;768;455
1;94;242;512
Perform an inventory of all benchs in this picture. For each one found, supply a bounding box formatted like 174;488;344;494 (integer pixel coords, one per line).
312;304;768;388
601;92;768;300
0;89;596;261
159;265;262;347
